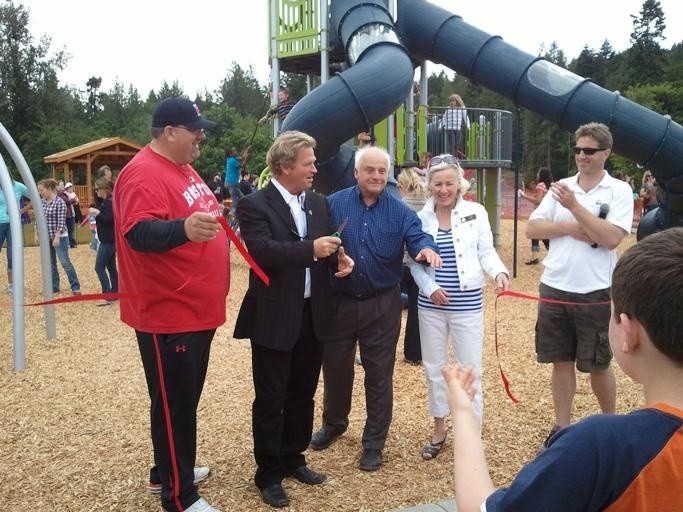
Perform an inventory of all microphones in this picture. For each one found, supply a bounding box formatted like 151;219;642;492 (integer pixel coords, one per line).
591;204;609;248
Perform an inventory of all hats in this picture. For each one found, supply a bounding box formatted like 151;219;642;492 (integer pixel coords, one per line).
153;99;217;131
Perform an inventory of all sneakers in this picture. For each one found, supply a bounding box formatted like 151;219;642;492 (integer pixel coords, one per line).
525;258;539;265
147;428;382;512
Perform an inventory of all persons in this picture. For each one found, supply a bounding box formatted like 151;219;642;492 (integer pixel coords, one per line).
306;145;444;472
258;88;298;133
1;166;33;294
409;151;512;462
437;225;683;512
55;179;80;248
617;169;659;234
438;93;470;159
384;151;434;363
94;165;115;209
467;177;477;192
517;184;526;209
206;142;259;249
233;129;356;508
524;121;637;432
87;203;98;250
519;167;554;264
34;179;81;296
87;176;118;306
110;96;234;512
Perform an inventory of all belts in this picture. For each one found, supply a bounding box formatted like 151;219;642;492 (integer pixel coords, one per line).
347;289;383;301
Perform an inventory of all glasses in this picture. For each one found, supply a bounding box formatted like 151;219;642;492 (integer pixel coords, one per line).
572;146;607;156
429;156;458;165
290;206;308;240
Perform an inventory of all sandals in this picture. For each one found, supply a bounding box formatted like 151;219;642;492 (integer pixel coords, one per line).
421;431;448;459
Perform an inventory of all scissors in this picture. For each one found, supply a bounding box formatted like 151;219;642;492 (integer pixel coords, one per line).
330;216;349;238
